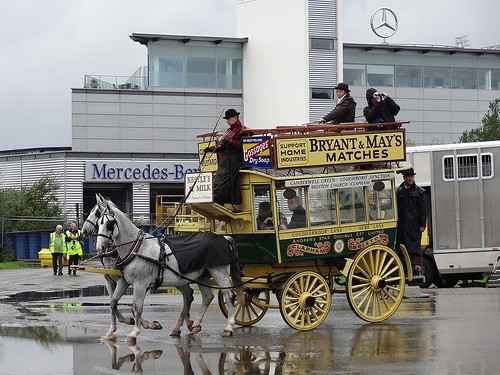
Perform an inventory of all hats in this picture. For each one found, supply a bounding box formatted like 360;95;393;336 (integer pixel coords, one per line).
221;107;240;120
332;82;351;91
401;166;416;176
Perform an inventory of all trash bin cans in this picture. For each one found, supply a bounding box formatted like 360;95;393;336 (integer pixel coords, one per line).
64;229;96;254
137;225;165;237
0;230;51;259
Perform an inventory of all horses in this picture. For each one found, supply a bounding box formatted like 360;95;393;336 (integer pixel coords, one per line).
96;202;245;342
81;193;194;340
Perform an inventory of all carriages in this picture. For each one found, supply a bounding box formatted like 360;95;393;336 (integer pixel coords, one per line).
69;119;428;345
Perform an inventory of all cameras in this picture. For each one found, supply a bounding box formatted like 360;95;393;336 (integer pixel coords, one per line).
378;93;384;97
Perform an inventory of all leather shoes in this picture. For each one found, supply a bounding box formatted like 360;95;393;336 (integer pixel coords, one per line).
53;272;63;275
67;272;77;276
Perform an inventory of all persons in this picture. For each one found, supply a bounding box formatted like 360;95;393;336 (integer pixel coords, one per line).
280;196;307;229
49;224;66;275
317;83;356;131
369;168;427;276
256;201;272;230
363;88;400;130
204;109;253;206
65;223;84;276
